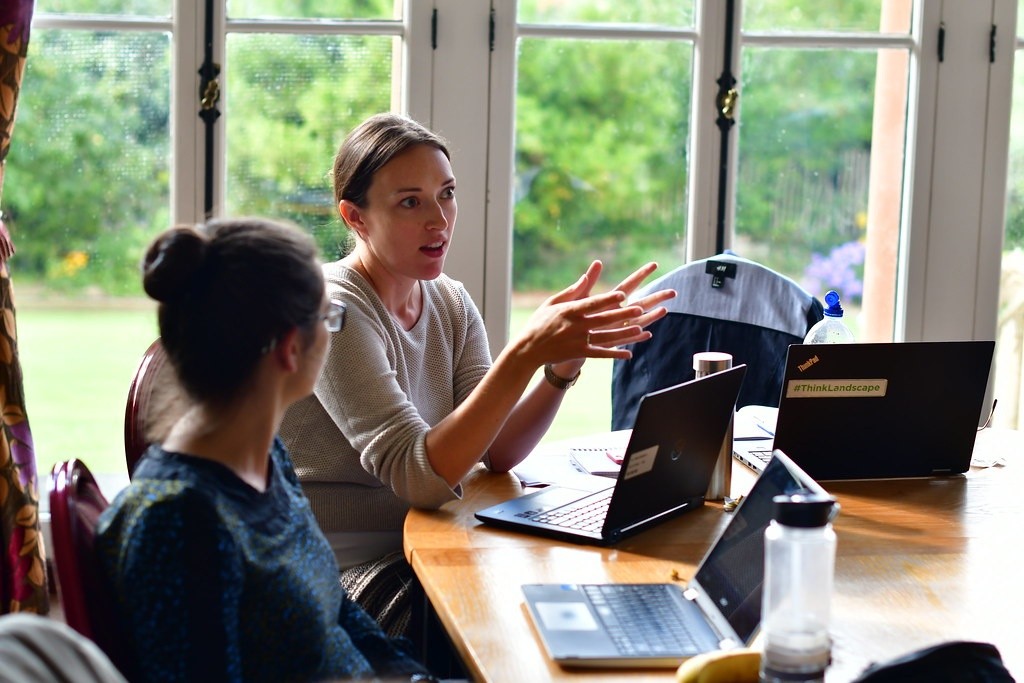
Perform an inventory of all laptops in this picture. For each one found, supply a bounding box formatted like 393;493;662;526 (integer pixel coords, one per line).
474;364;748;547
732;340;996;482
521;449;841;668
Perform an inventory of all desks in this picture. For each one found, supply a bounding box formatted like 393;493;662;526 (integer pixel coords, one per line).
402;431;1024;683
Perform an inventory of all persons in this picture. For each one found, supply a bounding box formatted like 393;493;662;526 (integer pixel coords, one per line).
277;109;676;680
0;0;52;616
94;215;444;683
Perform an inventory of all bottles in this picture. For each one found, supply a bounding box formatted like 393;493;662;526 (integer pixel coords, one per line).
762;492;836;674
693;351;736;500
803;290;855;344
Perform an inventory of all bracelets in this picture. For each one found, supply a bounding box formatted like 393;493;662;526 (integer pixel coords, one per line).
543;362;580;389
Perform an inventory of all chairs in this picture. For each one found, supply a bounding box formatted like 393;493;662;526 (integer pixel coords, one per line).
48;458;114;638
612;251;832;433
125;337;179;488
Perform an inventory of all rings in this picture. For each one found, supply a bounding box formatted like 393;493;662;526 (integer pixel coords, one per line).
623;320;629;327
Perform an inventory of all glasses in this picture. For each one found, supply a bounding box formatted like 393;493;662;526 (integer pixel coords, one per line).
303;299;348;333
